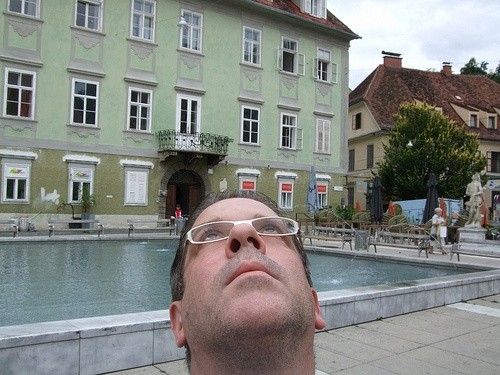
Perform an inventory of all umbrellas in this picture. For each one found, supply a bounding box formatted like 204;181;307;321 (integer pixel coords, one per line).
422;173;439;224
370;176;384;225
306;166;320;213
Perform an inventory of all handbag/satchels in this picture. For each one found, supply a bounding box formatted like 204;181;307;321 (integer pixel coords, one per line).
439;226;447;237
430;233;437;240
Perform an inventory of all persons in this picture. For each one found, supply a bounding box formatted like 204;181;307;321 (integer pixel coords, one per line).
429;207;448;254
464;175;484;224
171;204;184;224
169;189;326;375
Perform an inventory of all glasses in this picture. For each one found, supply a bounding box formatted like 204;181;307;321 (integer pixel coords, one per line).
181;217;302;262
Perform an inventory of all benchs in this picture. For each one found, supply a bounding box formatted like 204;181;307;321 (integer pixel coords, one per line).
0;218;19;238
450;238;500;262
301;225;353;251
367;231;433;258
47;219;105;238
126;217;177;236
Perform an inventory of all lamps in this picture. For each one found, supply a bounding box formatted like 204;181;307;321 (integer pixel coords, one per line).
268;165;270;169
226;161;228;165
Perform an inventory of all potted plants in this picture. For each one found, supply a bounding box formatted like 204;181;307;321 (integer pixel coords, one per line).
81;186;96;233
